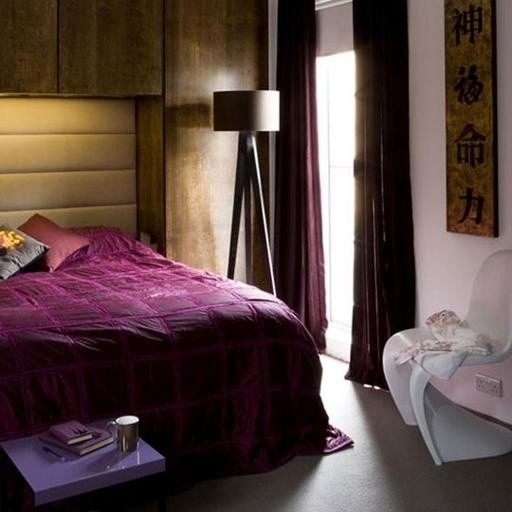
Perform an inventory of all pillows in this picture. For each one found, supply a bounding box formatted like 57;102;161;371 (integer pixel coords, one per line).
0;225;49;280
16;213;91;274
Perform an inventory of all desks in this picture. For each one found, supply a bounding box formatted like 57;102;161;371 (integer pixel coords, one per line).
0;417;166;506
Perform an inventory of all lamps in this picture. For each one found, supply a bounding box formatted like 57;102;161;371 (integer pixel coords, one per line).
212;90;281;298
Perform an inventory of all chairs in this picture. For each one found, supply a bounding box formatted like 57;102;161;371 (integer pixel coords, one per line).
382;249;512;466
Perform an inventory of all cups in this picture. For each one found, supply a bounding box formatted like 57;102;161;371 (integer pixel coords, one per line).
105;415;140;453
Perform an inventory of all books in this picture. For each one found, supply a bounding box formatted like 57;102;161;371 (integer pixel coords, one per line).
39;425;113;458
51;420;91;444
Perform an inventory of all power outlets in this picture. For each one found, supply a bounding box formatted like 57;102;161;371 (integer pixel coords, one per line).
473;373;503;397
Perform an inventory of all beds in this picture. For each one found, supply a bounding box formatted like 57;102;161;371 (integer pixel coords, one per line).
0;226;354;472
